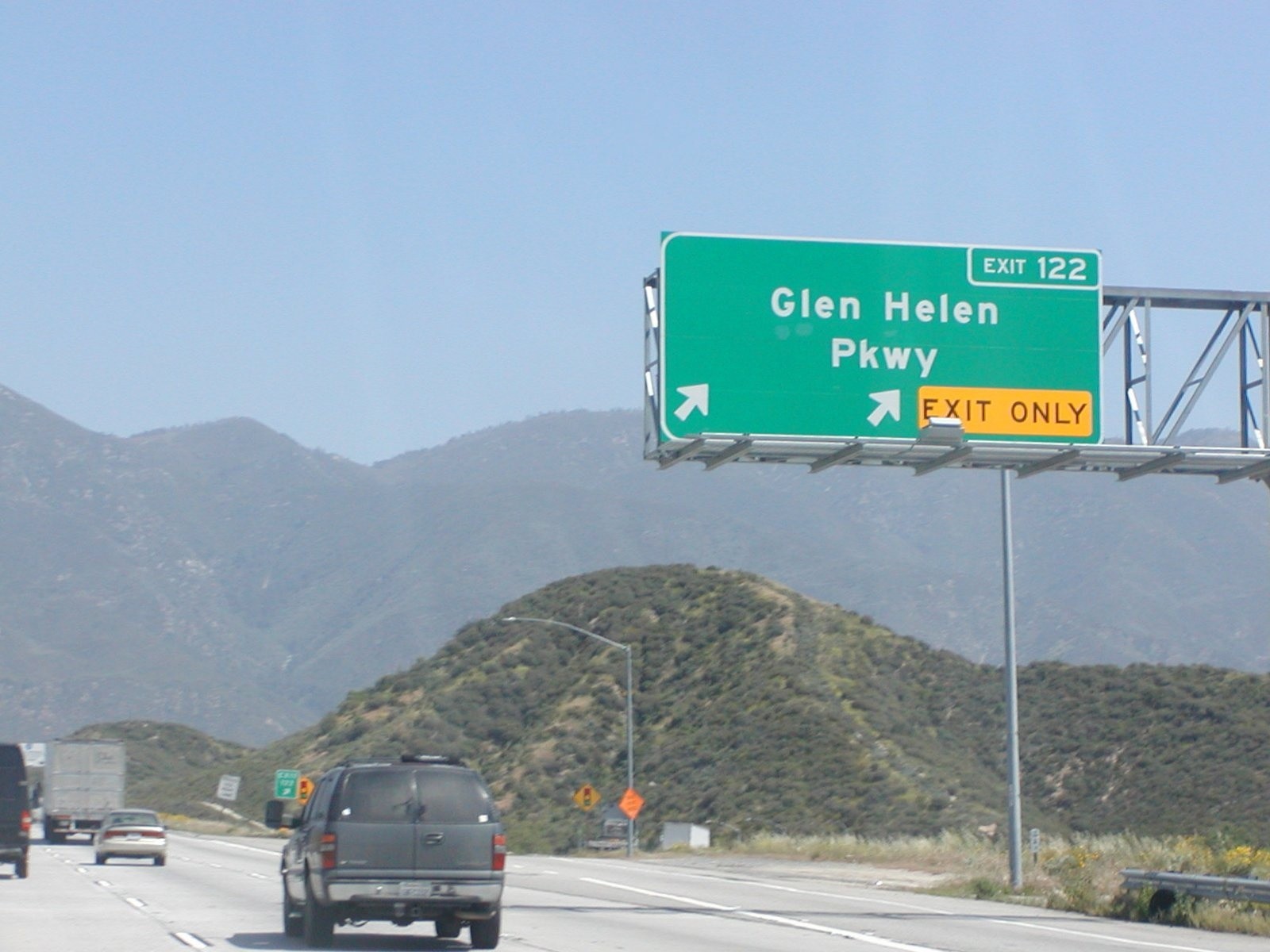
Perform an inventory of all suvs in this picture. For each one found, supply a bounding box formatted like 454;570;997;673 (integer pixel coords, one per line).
265;754;508;949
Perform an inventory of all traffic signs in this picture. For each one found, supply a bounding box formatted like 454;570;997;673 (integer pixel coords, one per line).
657;228;1106;450
275;769;300;800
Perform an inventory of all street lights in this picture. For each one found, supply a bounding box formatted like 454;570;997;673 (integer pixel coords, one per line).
500;616;636;860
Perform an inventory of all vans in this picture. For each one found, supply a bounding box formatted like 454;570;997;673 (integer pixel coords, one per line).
0;742;40;878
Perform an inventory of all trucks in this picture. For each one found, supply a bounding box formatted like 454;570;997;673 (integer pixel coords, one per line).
37;738;127;844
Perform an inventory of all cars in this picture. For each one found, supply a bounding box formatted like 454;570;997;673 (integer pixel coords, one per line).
94;809;170;866
